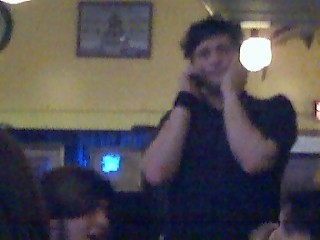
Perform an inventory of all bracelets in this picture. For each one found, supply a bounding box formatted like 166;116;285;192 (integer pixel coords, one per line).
174;91;198;111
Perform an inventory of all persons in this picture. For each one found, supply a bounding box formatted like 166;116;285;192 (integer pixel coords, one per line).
269;190;320;240
141;17;297;240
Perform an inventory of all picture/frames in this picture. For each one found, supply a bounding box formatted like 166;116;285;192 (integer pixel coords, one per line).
75;2;153;59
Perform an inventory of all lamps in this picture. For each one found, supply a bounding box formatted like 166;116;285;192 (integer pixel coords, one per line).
239;28;272;72
